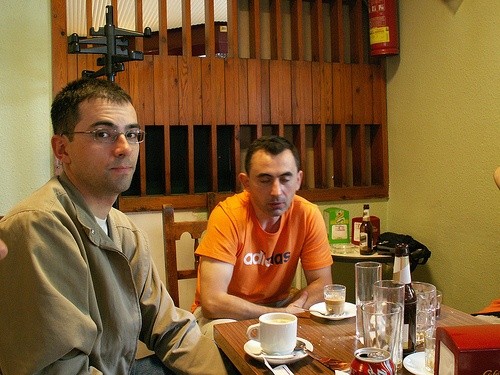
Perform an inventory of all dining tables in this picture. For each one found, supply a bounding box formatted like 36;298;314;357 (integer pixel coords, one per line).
213;304;492;375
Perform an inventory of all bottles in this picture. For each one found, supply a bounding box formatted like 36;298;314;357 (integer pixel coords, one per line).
359;204;375;255
387;244;416;359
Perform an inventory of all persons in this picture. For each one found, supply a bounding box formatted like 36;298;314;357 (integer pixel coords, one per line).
471;166;500;324
0;78;227;375
190;136;333;346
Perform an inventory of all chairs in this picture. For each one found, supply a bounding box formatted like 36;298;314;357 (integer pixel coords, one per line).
163;204;208;308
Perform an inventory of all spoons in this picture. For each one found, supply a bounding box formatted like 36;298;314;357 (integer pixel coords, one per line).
294;304;337;317
295;340;344;371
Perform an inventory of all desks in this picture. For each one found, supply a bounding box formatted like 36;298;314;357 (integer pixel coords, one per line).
330;242;426;263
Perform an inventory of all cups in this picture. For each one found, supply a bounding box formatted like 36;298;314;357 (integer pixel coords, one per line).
422;328;435;373
323;284;346;313
372;279;406;369
354;262;383;349
436;290;442;320
409;281;436;349
360;303;404;372
246;312;298;356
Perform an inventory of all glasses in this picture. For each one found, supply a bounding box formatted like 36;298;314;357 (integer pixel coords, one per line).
60;127;147;144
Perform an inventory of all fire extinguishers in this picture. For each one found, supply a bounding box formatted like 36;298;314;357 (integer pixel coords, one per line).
368;0;399;56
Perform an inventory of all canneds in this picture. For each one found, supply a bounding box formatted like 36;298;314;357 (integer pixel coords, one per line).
350;347;395;375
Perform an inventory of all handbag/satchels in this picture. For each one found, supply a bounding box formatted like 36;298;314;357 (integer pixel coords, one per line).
378;232;432;274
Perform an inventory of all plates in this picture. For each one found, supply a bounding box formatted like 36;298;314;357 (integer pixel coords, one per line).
402;351;434;375
244;336;313;367
310;301;357;320
